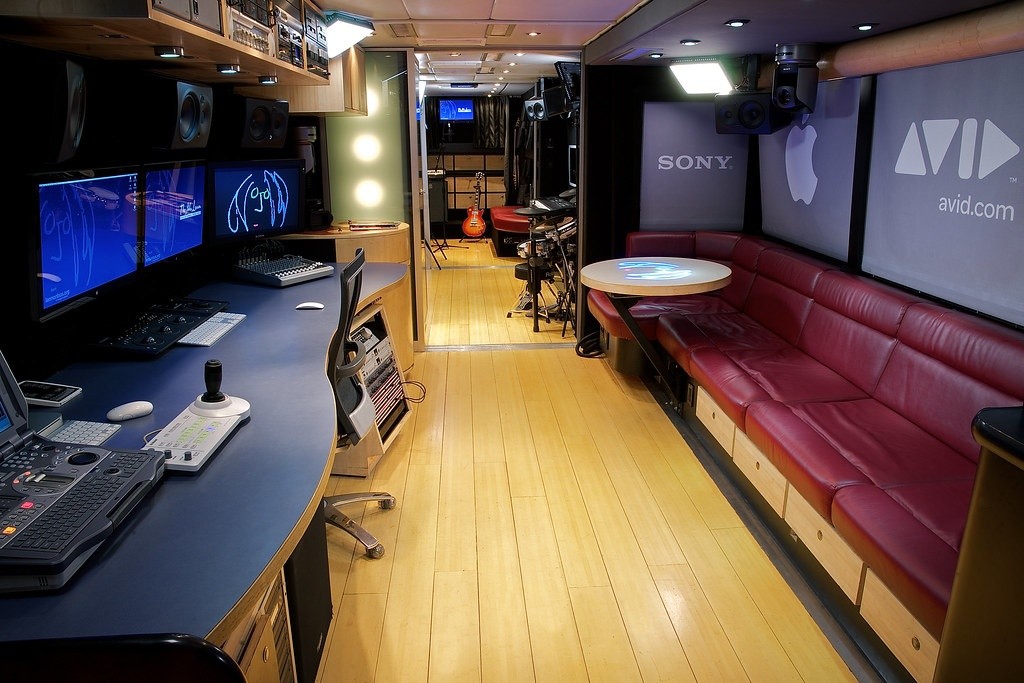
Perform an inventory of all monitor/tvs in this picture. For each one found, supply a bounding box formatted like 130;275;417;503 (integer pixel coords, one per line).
438;97;474;124
568;145;577;187
26;157;307;322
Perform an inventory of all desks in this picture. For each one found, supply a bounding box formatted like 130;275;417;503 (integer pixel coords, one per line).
0;261;411;683
580;256;732;402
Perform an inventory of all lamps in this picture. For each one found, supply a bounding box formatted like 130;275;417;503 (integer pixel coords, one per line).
666;58;735;95
327;12;376;61
153;46;185;57
218;64;241;73
258;75;278;85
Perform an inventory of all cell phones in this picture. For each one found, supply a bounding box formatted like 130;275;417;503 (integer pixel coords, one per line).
18;380;83;407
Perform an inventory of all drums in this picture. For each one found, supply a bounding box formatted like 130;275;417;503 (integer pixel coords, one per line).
531;217;577;240
517;237;556;259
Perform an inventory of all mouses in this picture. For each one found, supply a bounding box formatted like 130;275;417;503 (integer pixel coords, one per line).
296;302;325;309
107;401;153;421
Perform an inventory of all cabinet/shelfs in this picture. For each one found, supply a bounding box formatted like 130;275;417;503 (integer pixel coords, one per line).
1;0;368;116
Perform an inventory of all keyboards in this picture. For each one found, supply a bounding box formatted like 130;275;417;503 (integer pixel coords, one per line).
177;312;247;346
46;419;121;446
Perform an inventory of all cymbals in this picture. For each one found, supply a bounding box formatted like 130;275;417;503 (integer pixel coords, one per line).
512;206;549;217
569;196;576;203
558;188;576;197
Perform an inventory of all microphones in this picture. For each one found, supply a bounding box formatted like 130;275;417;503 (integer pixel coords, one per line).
448;123;452;141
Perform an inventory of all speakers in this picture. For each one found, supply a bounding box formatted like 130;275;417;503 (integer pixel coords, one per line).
238;98;290;148
157;80;213;149
37;58;88;159
714;88;792;135
525;98;549;122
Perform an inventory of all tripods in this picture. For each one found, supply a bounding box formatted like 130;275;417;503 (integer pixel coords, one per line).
430;123;470;254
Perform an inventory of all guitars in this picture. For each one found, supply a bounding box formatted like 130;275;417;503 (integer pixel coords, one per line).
461;171;487;238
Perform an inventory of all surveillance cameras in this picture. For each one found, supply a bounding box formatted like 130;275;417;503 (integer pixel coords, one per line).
772;44;821;114
292;125;317;172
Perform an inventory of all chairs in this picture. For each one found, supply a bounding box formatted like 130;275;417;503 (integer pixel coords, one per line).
314;247;398;559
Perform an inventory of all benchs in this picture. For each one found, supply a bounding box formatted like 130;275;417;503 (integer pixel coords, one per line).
587;228;1024;683
489;206;530;258
